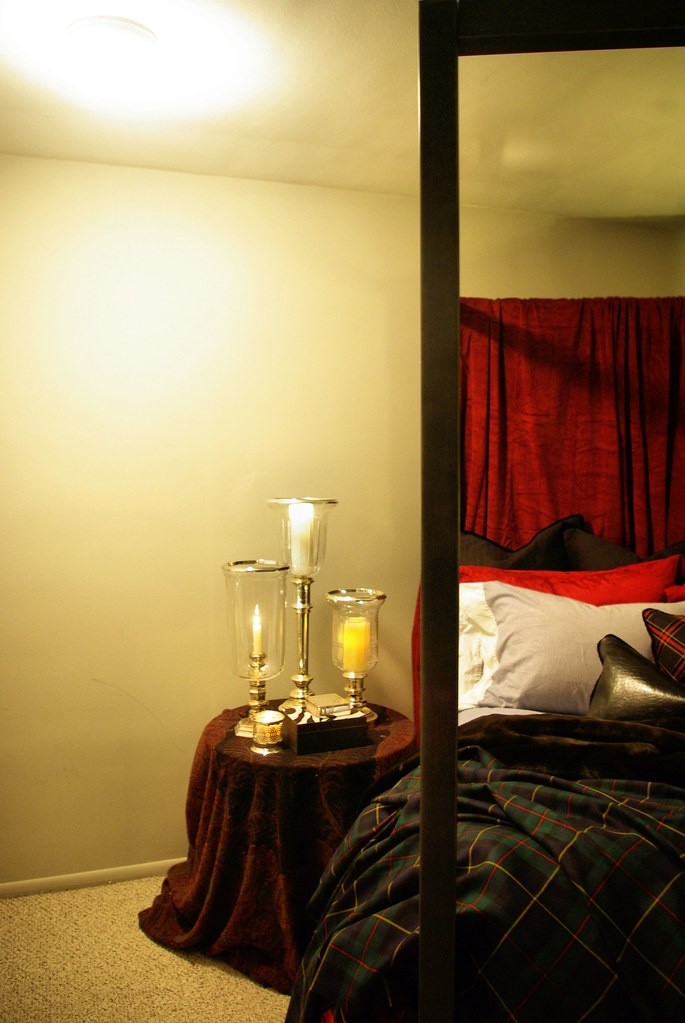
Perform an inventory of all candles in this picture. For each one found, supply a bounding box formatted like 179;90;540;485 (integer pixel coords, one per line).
251;603;265;655
341;617;371;673
288;498;315;573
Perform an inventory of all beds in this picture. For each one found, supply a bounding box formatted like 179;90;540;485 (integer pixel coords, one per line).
286;0;685;1023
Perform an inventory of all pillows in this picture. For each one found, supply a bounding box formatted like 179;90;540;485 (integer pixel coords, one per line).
587;633;685;721
460;553;682;607
641;608;685;686
480;582;685;715
460;513;587;570
565;529;685;585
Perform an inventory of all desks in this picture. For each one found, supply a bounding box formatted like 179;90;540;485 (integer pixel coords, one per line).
137;696;416;997
